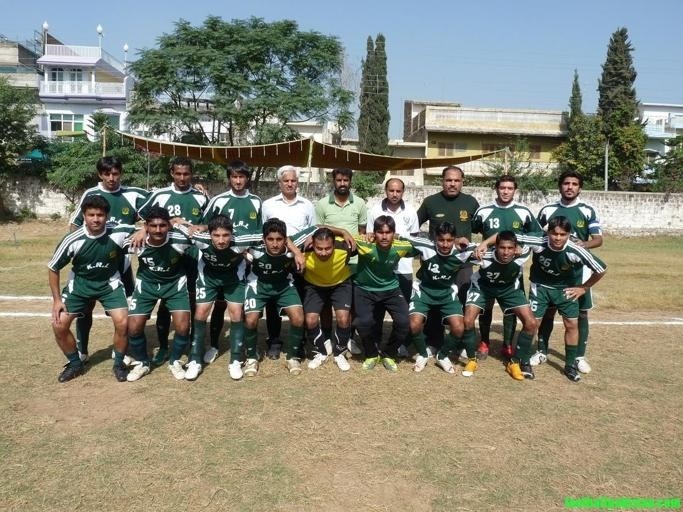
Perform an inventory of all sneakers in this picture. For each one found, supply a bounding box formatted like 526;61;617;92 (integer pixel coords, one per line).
112;347;219;382
59;351;89;382
565;356;591;381
227;339;490;381
502;345;546;380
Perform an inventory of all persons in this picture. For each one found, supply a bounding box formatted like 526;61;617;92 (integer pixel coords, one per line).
46;155;608;379
